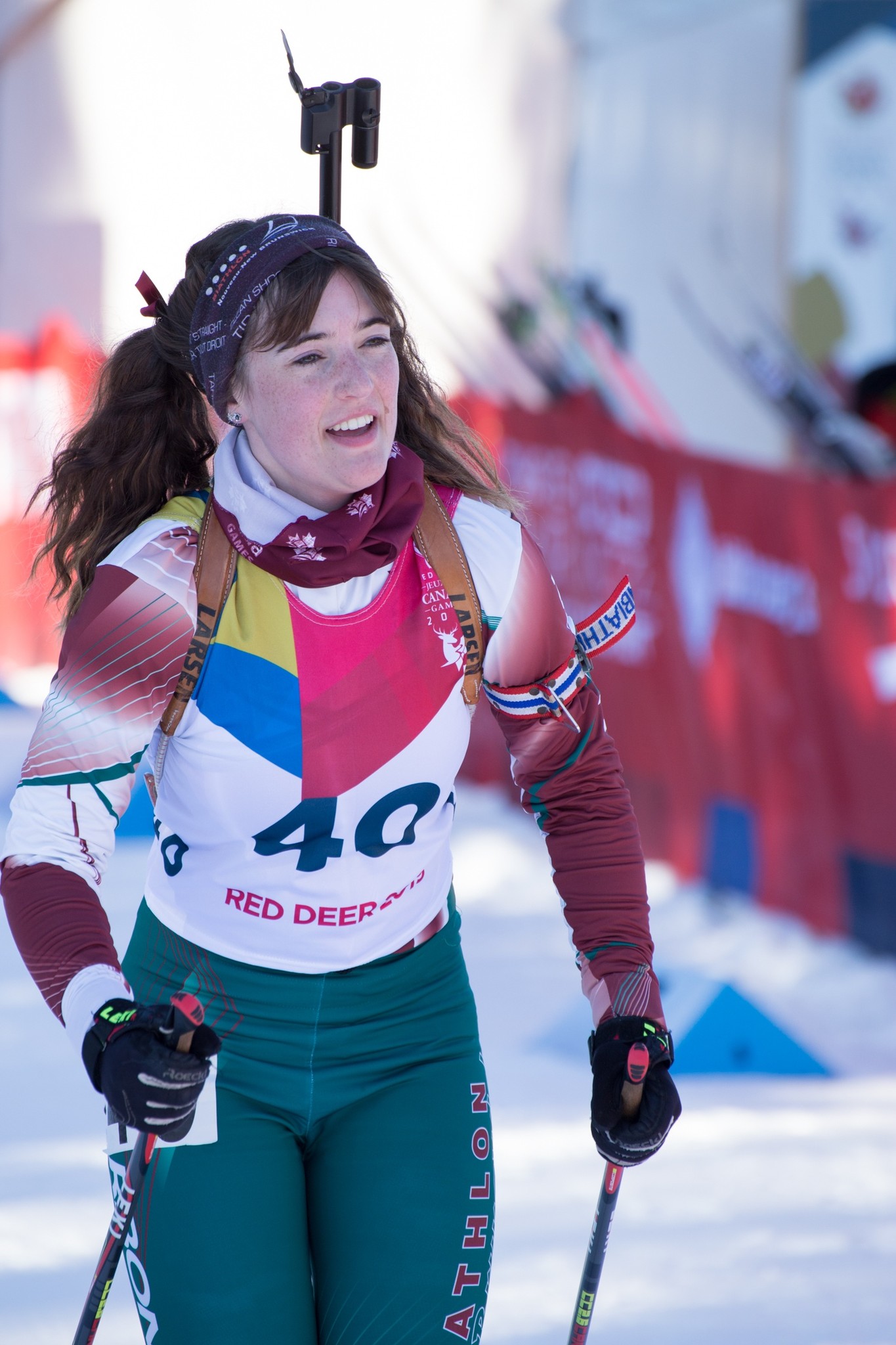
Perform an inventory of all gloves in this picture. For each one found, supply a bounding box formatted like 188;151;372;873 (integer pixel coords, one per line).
84;994;221;1143
590;1014;682;1163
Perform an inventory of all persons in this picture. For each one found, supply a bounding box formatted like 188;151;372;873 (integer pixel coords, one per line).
0;213;681;1345
